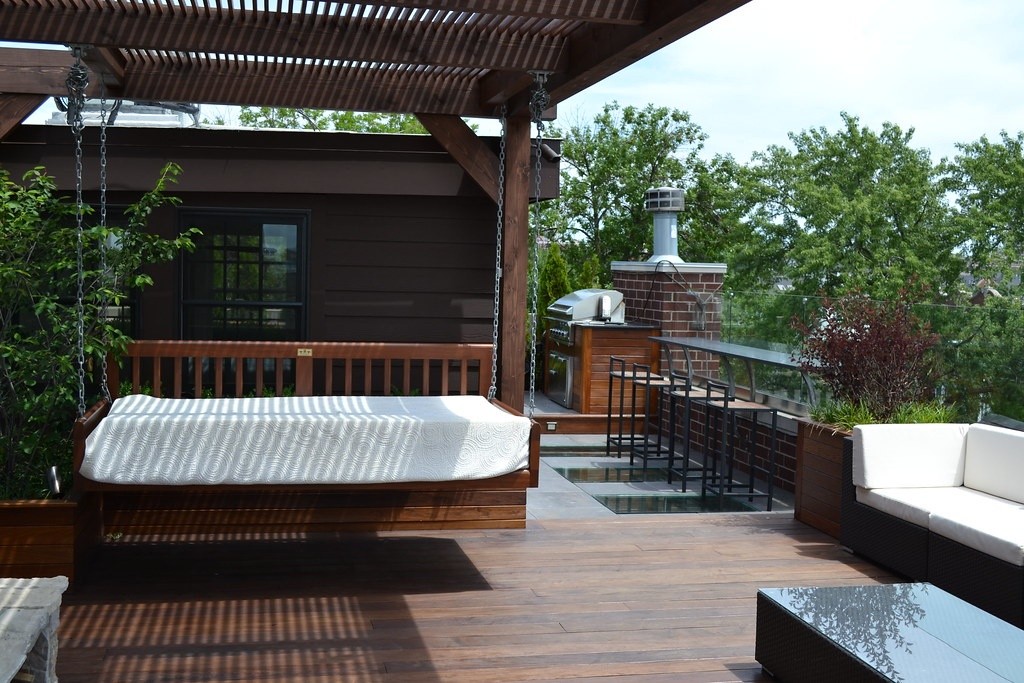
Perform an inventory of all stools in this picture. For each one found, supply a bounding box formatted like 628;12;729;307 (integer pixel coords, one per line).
629;362;691;467
605;355;664;459
701;380;780;513
667;371;736;494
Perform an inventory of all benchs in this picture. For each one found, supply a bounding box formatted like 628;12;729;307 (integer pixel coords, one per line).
70;341;543;537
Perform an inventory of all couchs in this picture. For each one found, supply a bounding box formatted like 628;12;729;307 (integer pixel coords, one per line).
837;421;1024;631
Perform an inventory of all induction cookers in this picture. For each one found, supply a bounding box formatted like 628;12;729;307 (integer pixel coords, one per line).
547;288;626;347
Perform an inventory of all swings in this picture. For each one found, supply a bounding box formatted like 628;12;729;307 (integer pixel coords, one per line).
65;62;550;529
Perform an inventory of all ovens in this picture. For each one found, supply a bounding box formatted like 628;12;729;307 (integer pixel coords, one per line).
546;351;572;409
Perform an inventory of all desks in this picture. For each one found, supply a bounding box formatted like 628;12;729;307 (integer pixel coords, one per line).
647;335;844;502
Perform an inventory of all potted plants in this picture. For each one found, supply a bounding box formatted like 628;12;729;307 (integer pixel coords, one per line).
791;272;960;542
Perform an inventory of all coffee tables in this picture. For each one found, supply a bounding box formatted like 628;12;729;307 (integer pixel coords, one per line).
754;579;1023;682
1;573;69;683
